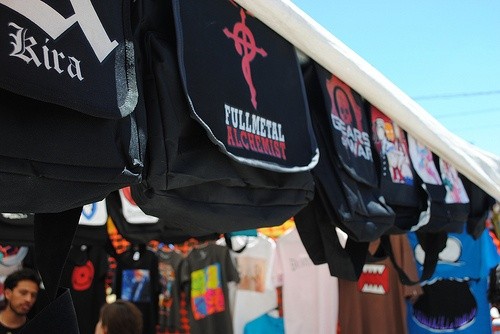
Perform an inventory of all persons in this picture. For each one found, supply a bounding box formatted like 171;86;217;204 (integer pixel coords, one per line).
1;268;41;334
93;301;143;334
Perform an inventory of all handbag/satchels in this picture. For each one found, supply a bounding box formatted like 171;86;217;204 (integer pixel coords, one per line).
109;0;315;243
437;157;474;241
294;57;395;285
0;1;140;221
402;130;452;282
362;105;432;256
